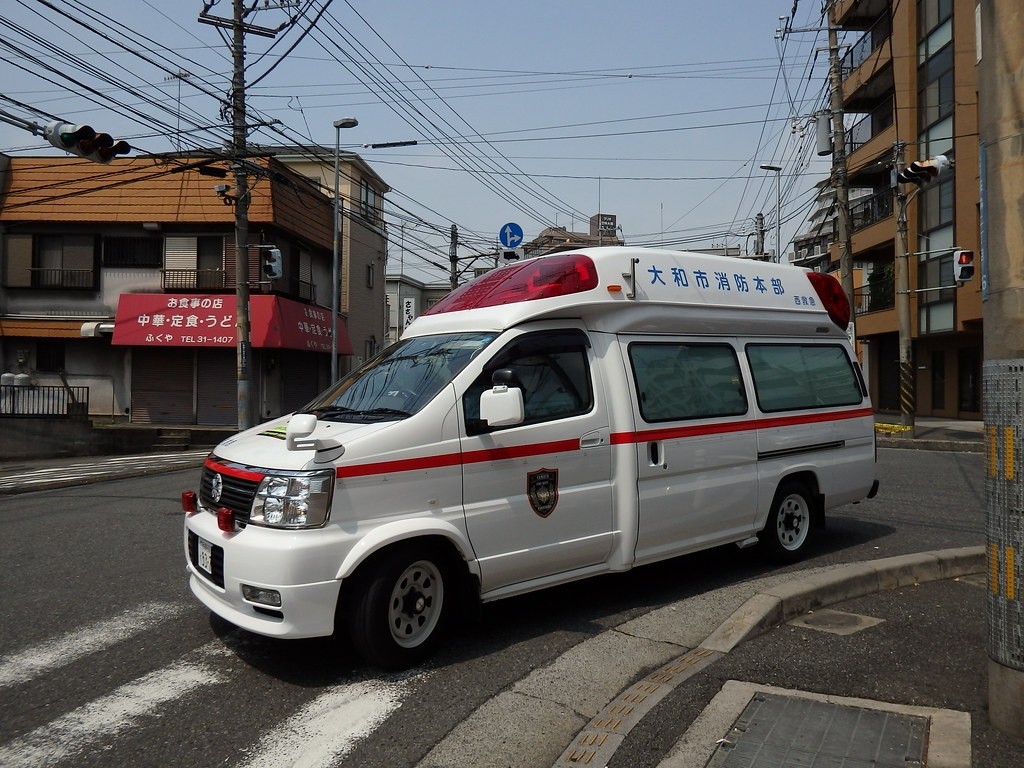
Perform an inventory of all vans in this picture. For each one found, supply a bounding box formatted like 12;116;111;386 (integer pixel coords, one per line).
182;247;880;672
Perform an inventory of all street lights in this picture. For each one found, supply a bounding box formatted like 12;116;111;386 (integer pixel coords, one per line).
330;115;361;386
760;163;783;265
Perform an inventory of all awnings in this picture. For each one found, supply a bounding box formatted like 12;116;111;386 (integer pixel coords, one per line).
110;293;238;347
249;294;356;355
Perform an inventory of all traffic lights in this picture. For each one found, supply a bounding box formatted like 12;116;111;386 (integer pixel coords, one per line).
896;154;950;187
46;120;132;165
263;249;283;279
953;250;976;284
